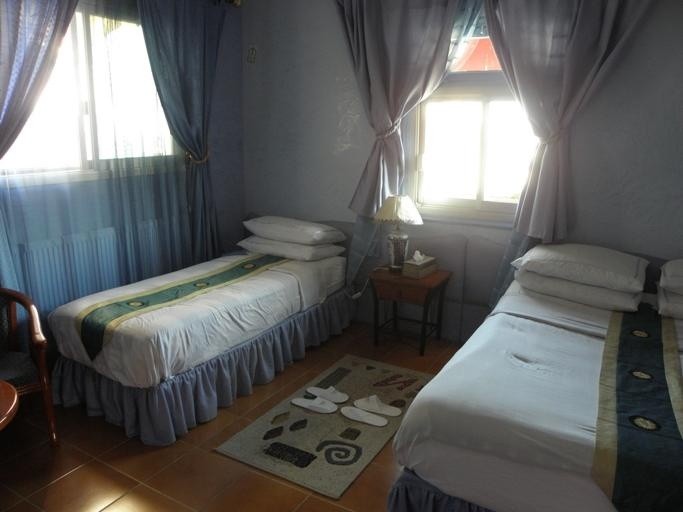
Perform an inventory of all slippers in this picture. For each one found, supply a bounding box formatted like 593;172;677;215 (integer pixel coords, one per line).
291;385;402;427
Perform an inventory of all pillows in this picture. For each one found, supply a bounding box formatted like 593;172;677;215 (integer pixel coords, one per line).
236;215;348;261
510;242;682;320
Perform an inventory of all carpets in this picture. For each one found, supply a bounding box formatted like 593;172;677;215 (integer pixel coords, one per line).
213;353;435;500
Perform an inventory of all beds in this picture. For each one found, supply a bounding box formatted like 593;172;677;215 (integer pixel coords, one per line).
385;280;682;512
46;250;358;446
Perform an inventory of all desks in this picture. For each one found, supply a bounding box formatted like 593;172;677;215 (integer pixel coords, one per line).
0;380;20;432
367;264;453;356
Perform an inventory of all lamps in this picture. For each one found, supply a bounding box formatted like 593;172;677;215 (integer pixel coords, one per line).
373;195;424;272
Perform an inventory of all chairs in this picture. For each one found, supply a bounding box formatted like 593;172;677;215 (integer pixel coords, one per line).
0;288;60;448
